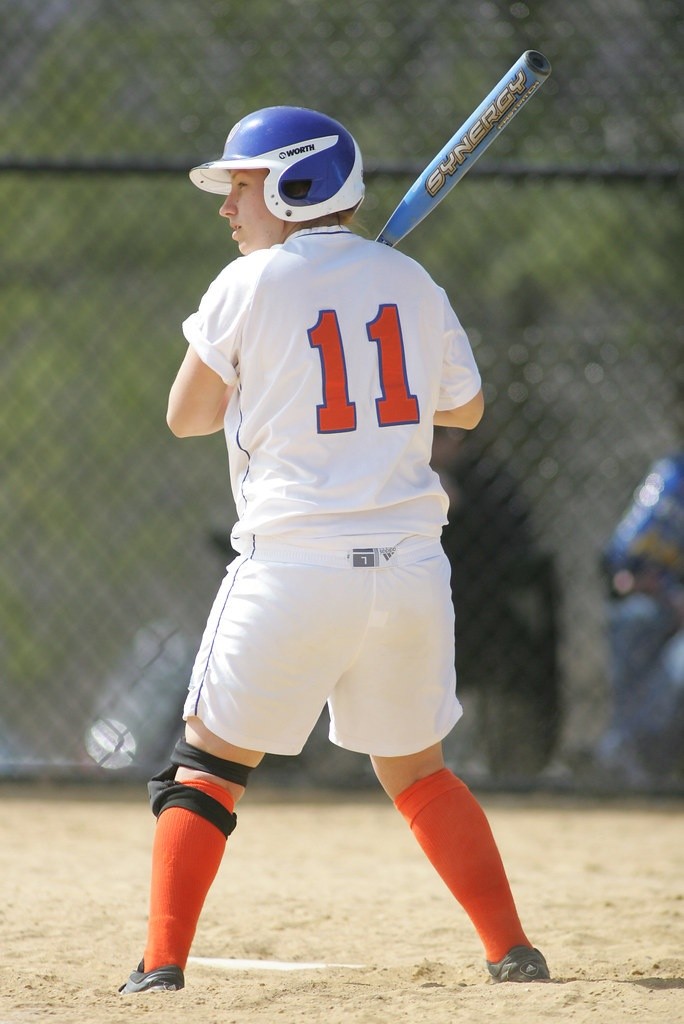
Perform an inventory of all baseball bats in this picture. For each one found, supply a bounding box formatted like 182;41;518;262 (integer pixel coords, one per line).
378;49;553;250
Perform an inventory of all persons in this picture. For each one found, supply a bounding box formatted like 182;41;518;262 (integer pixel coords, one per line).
113;104;551;989
427;425;683;801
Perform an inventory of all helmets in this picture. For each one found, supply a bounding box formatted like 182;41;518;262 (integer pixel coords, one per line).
189;106;365;222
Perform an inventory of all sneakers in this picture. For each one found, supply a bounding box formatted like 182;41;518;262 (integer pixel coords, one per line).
486;946;551;984
118;959;185;994
